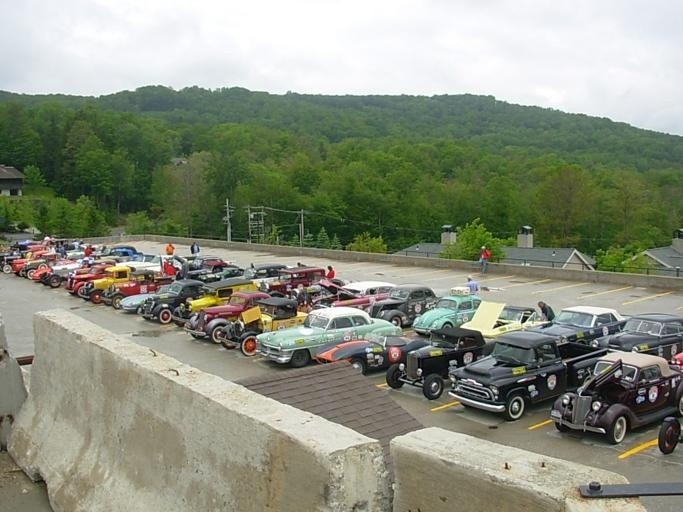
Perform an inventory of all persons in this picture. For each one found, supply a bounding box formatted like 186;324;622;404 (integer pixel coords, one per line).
327;265;335;279
466;275;480;295
165;242;175;255
479;246;491;273
538;301;555;327
48;238;107;259
191;242;200;263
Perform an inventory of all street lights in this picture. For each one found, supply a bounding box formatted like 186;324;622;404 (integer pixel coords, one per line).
171;157;192;239
